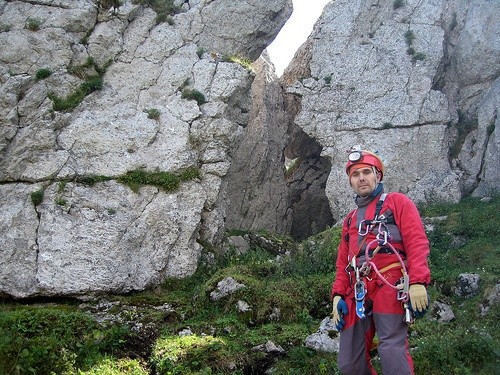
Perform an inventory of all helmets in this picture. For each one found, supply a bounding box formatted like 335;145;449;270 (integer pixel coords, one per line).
345;150;384;182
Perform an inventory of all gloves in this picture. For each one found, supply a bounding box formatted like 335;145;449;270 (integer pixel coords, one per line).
333;295;348;332
408;284;429;318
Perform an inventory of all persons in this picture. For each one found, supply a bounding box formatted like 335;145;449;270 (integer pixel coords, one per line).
334;150;429;375
